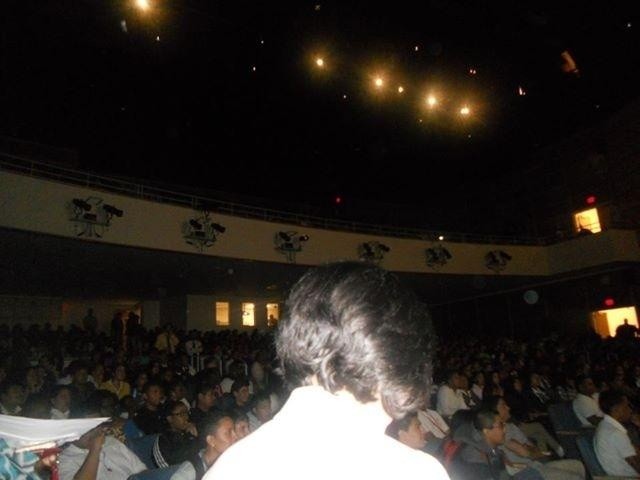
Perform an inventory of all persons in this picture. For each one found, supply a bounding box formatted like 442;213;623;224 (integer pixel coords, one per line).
0;261;640;480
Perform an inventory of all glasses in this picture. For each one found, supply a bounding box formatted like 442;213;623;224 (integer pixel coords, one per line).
170;410;193;417
